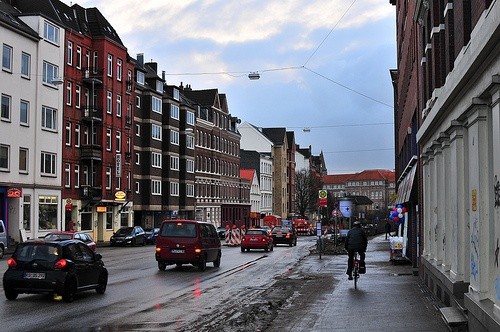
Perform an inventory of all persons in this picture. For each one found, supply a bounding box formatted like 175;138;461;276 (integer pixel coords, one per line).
344;221;368;280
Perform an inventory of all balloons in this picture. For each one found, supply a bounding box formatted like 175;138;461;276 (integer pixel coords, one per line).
389;205;407;225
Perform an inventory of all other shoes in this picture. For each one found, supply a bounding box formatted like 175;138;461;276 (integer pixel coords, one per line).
348;277;353;280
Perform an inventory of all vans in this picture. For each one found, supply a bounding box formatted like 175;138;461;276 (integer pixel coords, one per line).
155;219;222;271
0;220;8;256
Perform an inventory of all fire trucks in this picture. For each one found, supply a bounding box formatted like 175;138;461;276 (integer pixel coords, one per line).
294;218;309;235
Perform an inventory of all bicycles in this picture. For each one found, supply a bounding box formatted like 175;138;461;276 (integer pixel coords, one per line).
353;251;359;288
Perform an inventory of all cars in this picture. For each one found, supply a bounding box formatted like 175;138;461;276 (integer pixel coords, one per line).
269;227;297;246
109;226;160;246
240;228;273;253
2;239;108;302
216;227;231;238
44;231;96;252
